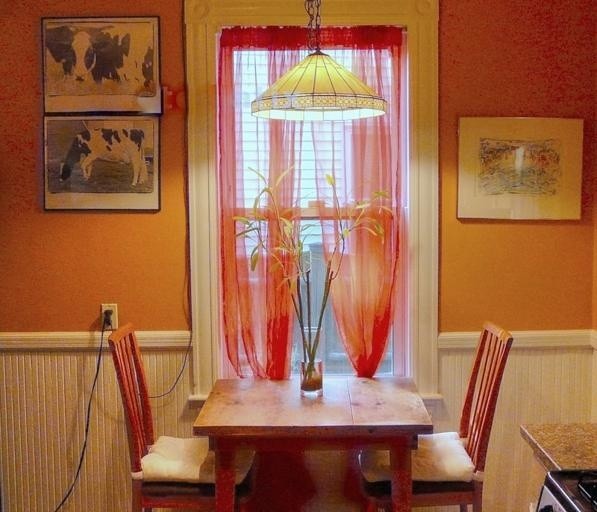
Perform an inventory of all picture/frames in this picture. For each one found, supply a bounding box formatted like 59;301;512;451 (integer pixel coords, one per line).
455;114;585;222
40;15;163;214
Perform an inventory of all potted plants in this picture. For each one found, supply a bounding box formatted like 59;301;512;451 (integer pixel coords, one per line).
230;163;397;398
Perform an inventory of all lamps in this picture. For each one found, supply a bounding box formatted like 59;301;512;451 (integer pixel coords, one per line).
251;1;387;122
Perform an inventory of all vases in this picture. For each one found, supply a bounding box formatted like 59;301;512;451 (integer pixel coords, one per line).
298;326;324;400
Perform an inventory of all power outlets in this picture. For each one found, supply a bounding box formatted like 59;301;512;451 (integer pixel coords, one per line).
101;303;119;330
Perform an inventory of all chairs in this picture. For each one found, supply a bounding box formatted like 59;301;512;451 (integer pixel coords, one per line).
103;322;256;512
354;317;515;511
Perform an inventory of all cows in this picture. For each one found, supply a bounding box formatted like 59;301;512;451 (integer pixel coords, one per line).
57;126;148;187
46;25;155;87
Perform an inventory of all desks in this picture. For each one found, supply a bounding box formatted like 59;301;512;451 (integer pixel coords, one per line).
194;379;433;511
